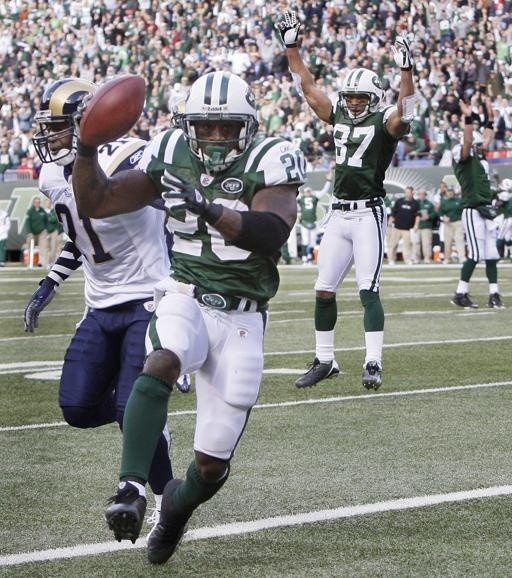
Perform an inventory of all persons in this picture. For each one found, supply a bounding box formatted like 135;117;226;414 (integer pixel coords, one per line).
70;72;308;562
386;185;418;266
410;189;442;265
24;197;48;244
297;188;319;255
494;178;511;255
427;189;467;265
271;0;418;15
0;0;272;72
417;0;512;112
23;79;174;542
1;74;40;180
416;110;446;169
273;8;417;388
446;99;505;314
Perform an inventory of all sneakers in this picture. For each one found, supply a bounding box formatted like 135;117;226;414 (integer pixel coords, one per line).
487;293;506;311
177;374;190;391
450;291;479;310
362;360;383;389
150;489;188;543
147;477;191;564
101;478;146;544
295;357;340;388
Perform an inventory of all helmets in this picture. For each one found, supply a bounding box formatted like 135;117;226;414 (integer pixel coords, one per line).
180;70;259;172
460;130;485;158
337;69;386;123
32;78;99;166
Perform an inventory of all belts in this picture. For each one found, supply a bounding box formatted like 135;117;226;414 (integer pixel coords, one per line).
166;279;270;313
322;199;384;212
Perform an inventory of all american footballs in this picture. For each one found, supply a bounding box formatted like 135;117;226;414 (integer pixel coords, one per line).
79;73;146;146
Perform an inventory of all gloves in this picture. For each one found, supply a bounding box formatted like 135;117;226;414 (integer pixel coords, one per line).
390;37;413;71
23;278;57;332
160;166;225;227
71;92;92;141
276;10;301;48
